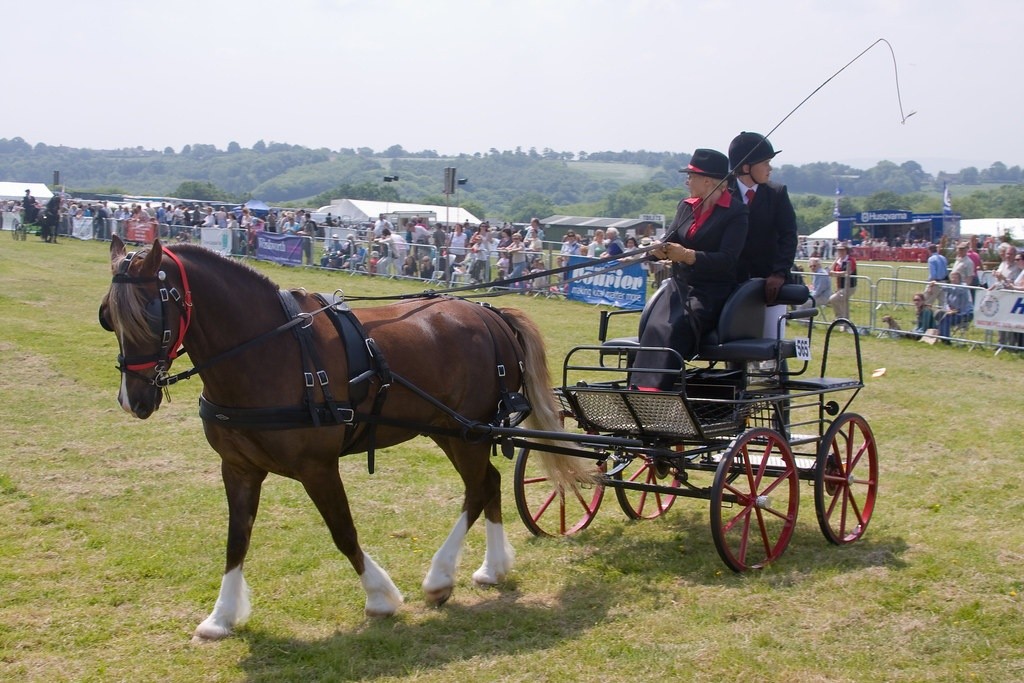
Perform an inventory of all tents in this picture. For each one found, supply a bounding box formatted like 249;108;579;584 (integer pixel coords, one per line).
309;199;482;228
232;199;271;220
0;182;54;204
808;218;1024;241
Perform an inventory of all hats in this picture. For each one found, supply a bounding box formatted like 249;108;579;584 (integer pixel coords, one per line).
679;148;739;179
957;242;969;249
638;238;654;248
836;243;847;249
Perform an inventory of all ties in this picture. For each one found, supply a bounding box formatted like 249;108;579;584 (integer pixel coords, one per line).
746;189;756;205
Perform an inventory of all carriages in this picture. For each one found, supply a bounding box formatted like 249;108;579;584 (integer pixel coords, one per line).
11;192;61;243
99;233;882;642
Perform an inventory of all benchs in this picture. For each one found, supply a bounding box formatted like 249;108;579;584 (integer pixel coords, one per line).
603;283;818;371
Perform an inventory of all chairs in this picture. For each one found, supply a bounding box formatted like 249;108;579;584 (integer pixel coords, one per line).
422;253;457;284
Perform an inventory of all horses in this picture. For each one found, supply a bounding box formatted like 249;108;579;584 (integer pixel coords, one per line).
95;232;610;648
36;193;62;244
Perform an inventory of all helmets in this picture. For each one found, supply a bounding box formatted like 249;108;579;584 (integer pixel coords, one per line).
728;131;781;170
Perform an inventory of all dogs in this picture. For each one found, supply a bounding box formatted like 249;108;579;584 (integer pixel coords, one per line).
882;314;901;334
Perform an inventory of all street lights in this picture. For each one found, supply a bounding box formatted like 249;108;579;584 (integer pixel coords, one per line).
456;178;468;223
384;174;400;224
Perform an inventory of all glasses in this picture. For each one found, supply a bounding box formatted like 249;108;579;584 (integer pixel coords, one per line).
568;235;574;237
513;238;518;240
1014;259;1023;261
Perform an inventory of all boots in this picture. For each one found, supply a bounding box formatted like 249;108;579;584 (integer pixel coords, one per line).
728;360;748;433
769;358;791;439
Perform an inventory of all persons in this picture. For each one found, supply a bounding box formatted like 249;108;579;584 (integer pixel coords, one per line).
797;236;1024;259
631;149;748;392
829;244;857;332
2;189;654;294
726;131;798;438
797;257;832;328
908;242;1024;351
